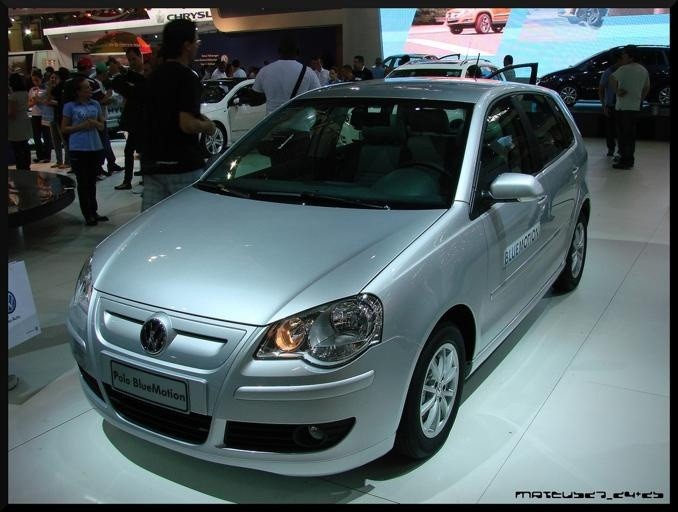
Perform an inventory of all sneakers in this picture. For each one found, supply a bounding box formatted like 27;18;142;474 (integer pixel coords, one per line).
33;158;50;163
51;164;70;169
607;149;633;169
85;212;109;226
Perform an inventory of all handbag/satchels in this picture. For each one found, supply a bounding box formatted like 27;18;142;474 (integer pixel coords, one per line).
8;260;41;349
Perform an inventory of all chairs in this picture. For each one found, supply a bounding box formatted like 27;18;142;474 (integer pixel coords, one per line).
396;109;464;168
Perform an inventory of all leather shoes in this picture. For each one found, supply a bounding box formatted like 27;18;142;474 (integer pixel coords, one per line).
134;171;144;176
96;167;108;181
114;183;132;190
107;163;125;171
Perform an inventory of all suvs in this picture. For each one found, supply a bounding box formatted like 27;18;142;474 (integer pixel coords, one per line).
446;8;510;34
337;58;507;145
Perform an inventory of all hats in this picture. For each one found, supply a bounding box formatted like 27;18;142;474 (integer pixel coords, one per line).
79;54;95;67
96;62;108;72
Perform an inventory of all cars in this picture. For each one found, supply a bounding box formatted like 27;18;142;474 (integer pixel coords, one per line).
131;78;266;156
372;53;438;77
65;74;590;477
539;45;670;106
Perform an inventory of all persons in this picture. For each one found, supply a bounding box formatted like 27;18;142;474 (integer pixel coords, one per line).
502;55;516;81
608;45;650;170
599;51;626;156
8;17;410;226
